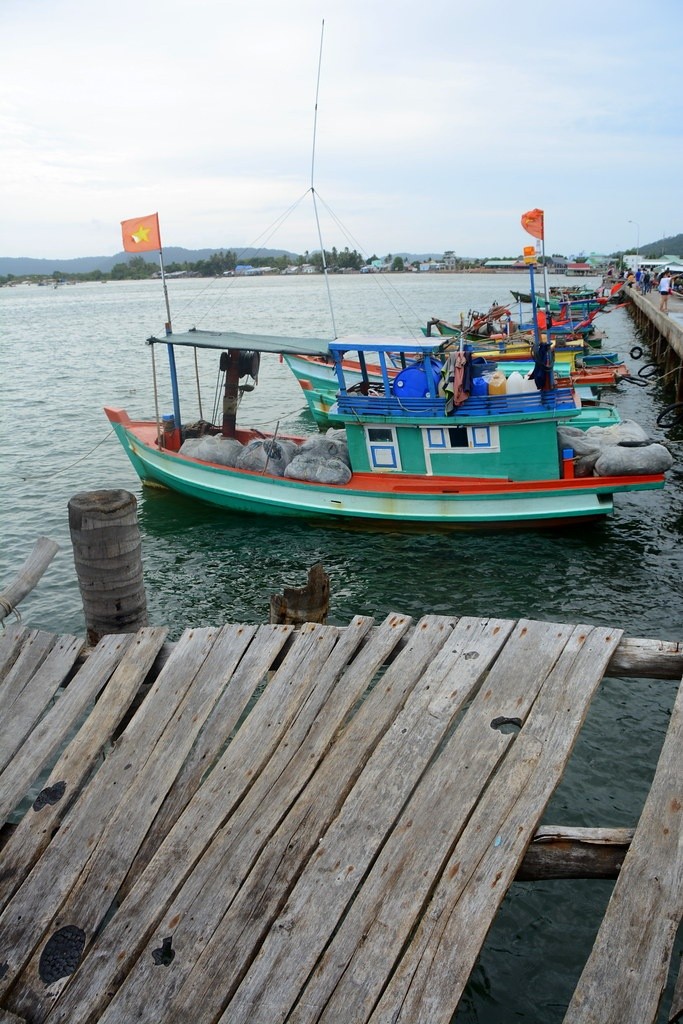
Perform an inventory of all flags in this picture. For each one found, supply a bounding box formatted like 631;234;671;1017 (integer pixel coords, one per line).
121;213;161;252
521;208;544;240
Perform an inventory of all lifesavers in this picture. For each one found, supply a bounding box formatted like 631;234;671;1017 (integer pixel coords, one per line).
637;363;659;377
630;347;643;360
656;402;683;429
635;328;648;339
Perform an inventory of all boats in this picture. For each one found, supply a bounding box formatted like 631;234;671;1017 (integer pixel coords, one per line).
282;282;634;432
104;14;670;536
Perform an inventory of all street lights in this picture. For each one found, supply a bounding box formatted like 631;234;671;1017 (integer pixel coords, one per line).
629;220;641;272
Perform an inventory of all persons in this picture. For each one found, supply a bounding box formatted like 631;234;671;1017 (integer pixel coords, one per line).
620;267;635;288
657;270;679;311
635;268;656;295
